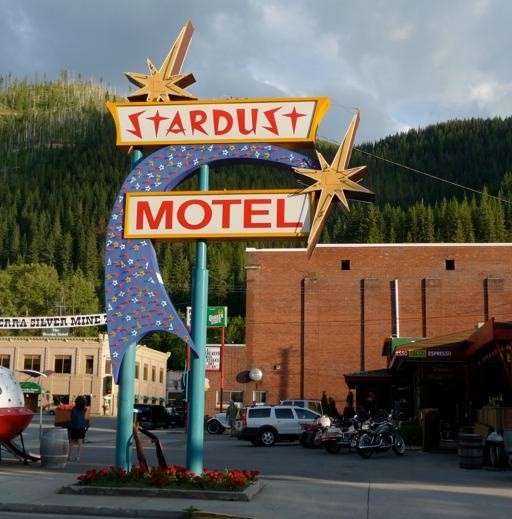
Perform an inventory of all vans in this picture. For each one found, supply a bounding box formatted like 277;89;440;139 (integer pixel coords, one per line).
237;399;323;446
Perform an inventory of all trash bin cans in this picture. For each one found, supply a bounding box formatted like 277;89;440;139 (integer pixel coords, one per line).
487;431;504;470
458;432;484;469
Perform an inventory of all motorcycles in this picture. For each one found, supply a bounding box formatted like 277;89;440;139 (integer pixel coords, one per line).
300;410;406;459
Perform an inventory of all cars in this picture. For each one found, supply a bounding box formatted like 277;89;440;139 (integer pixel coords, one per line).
206;412;231;434
49;406;56;415
134;404;184;429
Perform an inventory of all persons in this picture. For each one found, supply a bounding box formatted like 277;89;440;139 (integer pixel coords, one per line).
226;400;238;437
68;395;86;462
